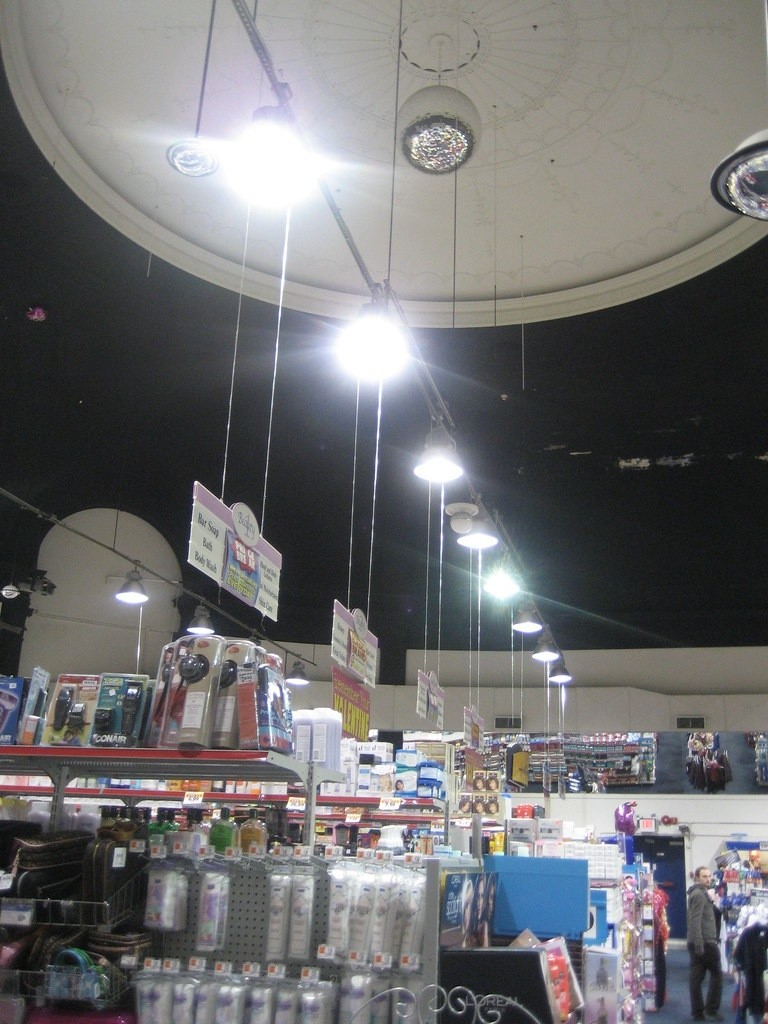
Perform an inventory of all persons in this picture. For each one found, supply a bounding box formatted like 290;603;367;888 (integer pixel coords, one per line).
459;873;495;948
687;866;721;1021
369;829;381;845
458;776;498;814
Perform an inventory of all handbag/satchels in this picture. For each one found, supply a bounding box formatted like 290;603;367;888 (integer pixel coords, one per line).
10;829;153;1008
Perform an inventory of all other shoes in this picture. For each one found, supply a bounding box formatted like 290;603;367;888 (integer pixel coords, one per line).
691;1010;724;1024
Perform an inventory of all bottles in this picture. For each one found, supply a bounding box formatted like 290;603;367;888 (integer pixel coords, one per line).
99;805;210;856
208;808;238;855
240;809;267;855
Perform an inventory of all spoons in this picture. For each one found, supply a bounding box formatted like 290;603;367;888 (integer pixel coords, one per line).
150;654;235;721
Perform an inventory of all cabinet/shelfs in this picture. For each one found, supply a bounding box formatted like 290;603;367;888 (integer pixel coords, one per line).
0;745;513;859
